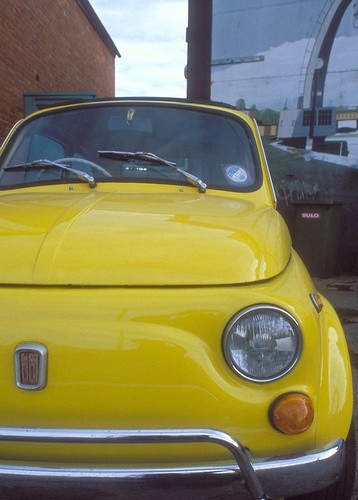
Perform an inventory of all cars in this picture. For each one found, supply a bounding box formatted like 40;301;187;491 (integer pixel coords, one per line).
0;95;356;499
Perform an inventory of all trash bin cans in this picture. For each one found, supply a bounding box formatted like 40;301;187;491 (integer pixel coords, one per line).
289;198;346;278
